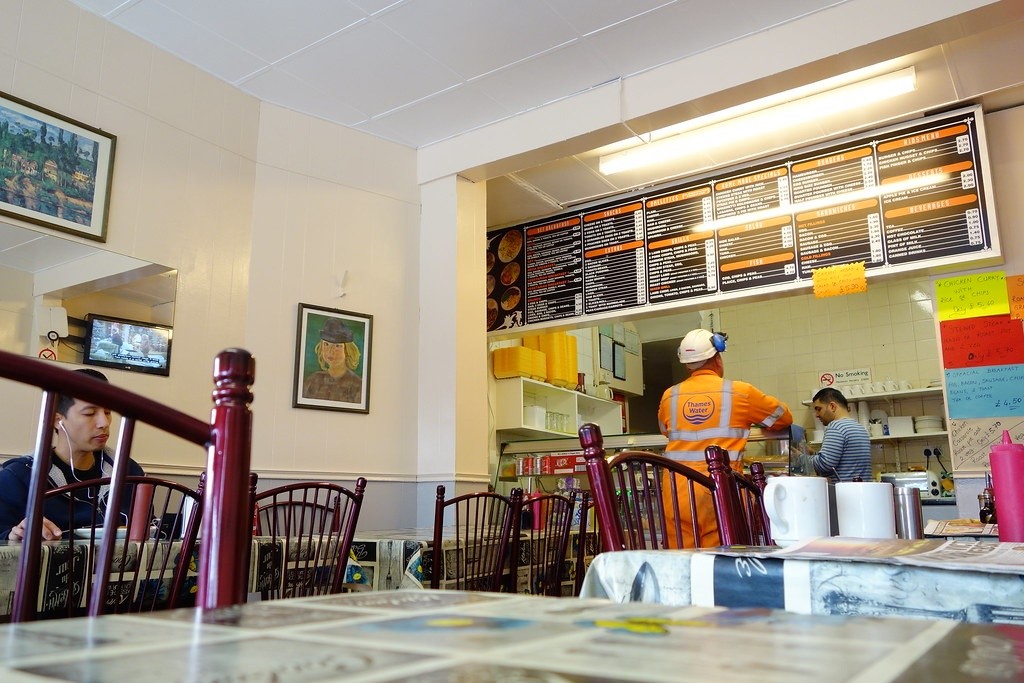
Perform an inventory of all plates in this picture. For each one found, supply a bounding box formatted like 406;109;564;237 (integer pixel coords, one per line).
73;527;127;539
914;415;944;433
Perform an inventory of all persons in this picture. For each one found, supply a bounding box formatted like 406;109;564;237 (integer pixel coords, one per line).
109;329;123;354
0;369;157;542
658;329;793;549
791;388;872;484
134;332;150;356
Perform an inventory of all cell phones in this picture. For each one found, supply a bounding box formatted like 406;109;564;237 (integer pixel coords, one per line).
153;513;182;539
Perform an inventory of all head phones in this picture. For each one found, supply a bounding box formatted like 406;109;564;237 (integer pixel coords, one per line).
712;331;728;352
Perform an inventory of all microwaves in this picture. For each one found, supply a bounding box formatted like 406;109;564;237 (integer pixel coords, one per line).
881;472;941;500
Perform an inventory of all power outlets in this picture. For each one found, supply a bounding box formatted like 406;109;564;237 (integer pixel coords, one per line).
921;446;944;457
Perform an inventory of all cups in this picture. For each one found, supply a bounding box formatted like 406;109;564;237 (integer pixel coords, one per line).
847;400;871;436
545;411;569;433
763;476;831;548
835;482;896;539
811;379;913;401
597;385;613;400
813;430;823;442
870;424;883;436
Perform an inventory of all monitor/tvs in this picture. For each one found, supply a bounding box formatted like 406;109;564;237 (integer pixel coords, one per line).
83;313;173;377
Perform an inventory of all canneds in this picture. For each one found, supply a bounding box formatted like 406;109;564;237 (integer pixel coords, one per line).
181;496;201;542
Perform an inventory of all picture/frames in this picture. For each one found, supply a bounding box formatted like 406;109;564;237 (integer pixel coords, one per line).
0;91;117;243
292;302;374;414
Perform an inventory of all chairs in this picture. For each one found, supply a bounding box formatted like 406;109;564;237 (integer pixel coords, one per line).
431;424;777;599
0;348;368;626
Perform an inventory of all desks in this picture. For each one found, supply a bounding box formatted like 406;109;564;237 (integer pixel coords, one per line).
0;524;1024;683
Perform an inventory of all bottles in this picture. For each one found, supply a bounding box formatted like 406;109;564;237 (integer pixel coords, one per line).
521;489;531;530
977;489;997;524
531;488;544;530
989;430;1024;542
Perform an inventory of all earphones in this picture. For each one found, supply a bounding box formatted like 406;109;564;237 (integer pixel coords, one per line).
59;421;66;433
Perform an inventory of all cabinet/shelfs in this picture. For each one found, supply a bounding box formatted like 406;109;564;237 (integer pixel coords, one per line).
496;376;623;439
487;422;820;549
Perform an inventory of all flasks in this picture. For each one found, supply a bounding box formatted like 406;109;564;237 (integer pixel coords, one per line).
893;487;924;540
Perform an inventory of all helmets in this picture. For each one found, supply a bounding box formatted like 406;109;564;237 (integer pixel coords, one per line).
678;329;716;363
